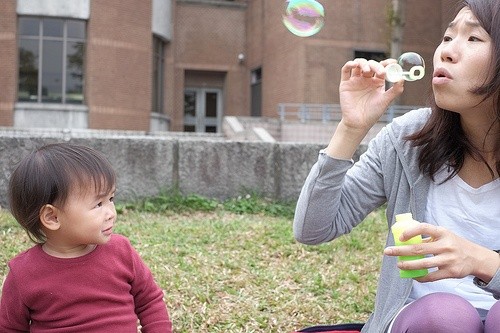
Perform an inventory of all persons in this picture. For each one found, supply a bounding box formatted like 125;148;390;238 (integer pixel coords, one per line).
293;0;500;333
0;143;172;333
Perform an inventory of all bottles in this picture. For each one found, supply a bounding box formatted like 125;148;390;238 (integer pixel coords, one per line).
390;213;429;278
